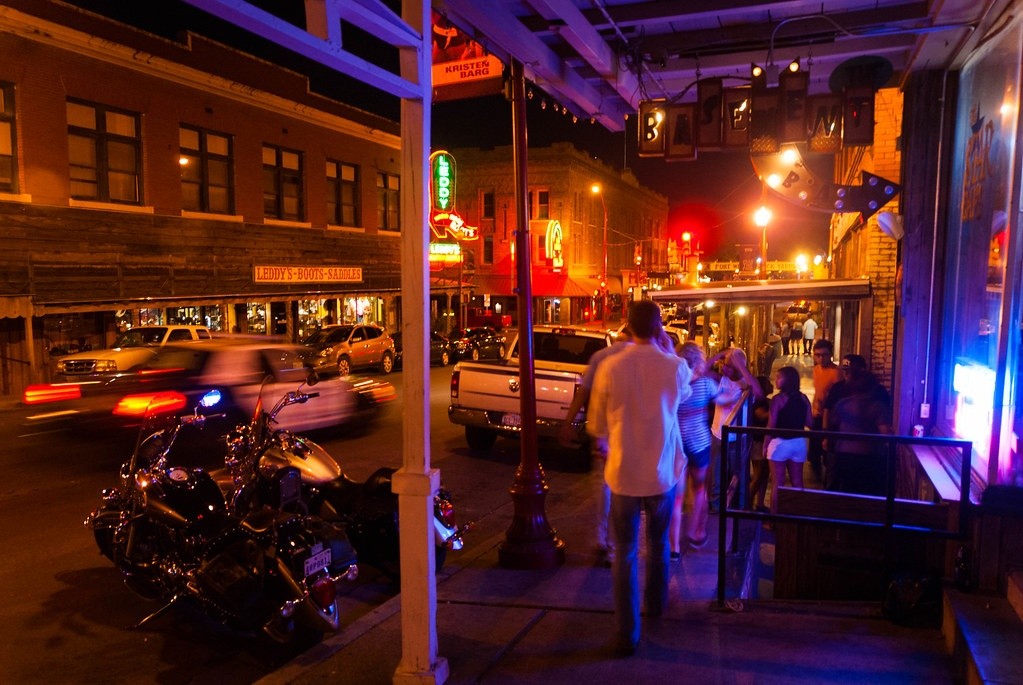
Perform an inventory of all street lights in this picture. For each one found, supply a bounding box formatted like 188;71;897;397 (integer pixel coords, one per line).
592;185;607;329
754;208;768;281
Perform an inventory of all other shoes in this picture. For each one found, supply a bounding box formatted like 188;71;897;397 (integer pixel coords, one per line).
591;545;612;570
670;551;680;562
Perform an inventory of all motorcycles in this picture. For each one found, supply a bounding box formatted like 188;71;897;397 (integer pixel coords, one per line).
85;390;359;657
216;370;475;593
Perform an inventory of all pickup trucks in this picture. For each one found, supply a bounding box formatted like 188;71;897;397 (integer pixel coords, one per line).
448;324;612;470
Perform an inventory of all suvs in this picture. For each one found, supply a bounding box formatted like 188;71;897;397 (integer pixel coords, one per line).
447;327;507;361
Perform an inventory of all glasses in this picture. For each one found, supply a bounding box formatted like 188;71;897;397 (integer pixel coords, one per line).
814;352;829;358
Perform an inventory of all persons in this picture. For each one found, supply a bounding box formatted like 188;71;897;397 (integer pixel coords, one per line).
586;300;692;656
559;321;893;569
781;312;818;355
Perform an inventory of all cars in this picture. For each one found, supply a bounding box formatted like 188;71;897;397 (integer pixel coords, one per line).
53;326;373;437
616;320;721;354
389;330;452;370
299;324;395;379
